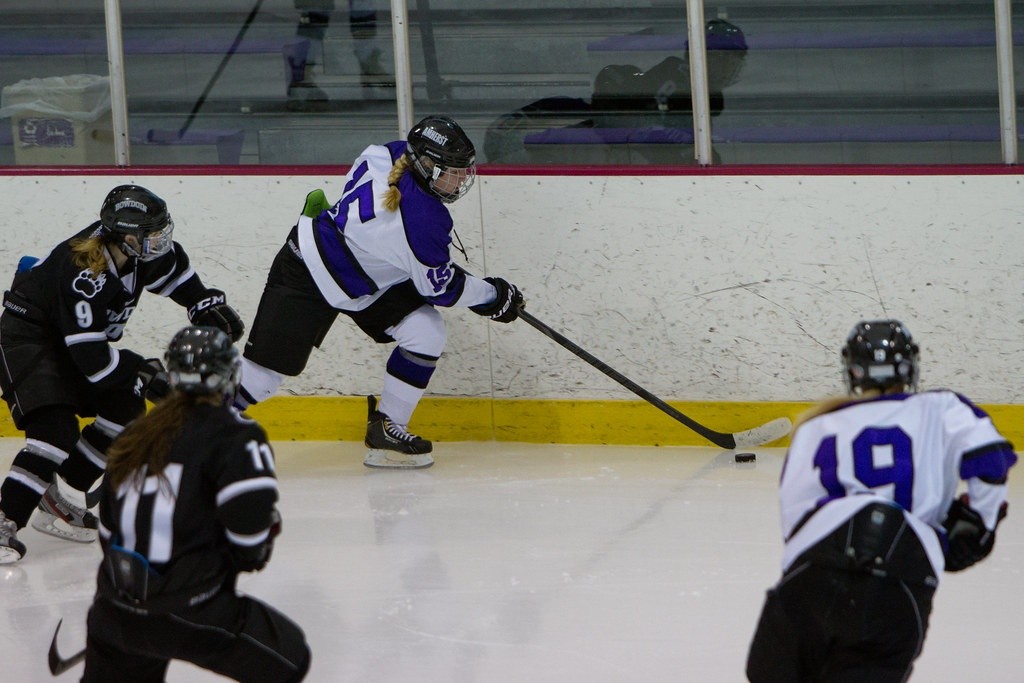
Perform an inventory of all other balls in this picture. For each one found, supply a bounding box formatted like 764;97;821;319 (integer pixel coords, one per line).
734;453;756;462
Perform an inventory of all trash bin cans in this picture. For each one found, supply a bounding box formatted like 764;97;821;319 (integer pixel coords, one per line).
3;73;116;166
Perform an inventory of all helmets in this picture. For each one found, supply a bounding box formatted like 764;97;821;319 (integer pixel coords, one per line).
406;114;476;204
164;326;243;401
839;321;920;394
702;20;748;50
591;65;647;98
103;184;171;262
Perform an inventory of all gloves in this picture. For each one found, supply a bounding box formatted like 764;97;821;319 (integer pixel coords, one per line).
187;288;245;343
941;494;1007;571
128;358;173;404
467;277;526;323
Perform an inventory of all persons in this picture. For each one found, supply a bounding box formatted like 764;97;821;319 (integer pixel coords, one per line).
747;318;1018;683
0;184;244;565
79;327;312;683
235;115;526;468
485;19;746;162
287;0;395;114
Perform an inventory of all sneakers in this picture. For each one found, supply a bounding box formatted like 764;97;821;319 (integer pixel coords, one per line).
30;472;99;542
0;510;27;564
361;74;398;100
363;395;434;467
286;81;331;109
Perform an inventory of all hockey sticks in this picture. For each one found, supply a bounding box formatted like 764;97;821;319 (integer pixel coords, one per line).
48;617;86;677
450;262;793;450
147;0;263;145
